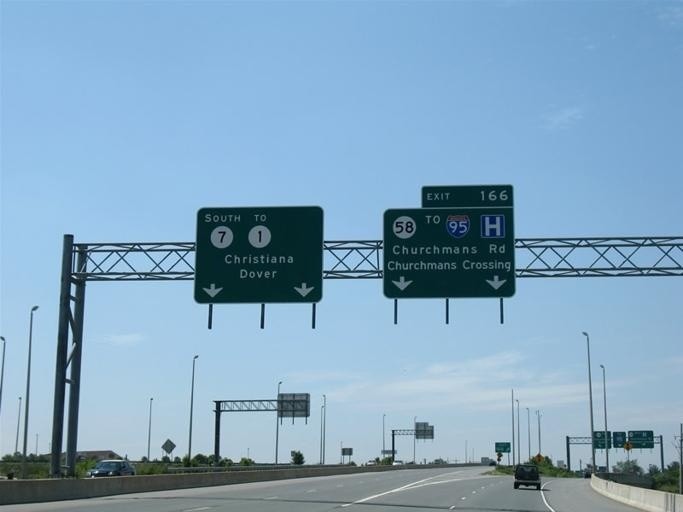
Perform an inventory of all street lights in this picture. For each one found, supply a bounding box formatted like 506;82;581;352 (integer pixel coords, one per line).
516;400;520;464
536;409;543;454
382;414;386;458
276;381;282;464
24;306;39;456
599;365;609;473
582;332;596;474
14;397;22;452
189;355;199;460
320;395;326;464
0;336;5;406
526;407;530;459
146;398;154;462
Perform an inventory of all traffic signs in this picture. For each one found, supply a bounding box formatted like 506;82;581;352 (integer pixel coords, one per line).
384;209;514;297
494;442;510;452
594;431;611;449
628;431;654;448
194;207;323;304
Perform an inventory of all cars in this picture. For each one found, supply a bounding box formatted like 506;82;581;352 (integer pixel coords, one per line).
392;461;403;466
367;460;376;465
87;460;136;476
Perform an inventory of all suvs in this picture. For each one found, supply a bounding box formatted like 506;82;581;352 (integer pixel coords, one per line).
513;465;541;490
